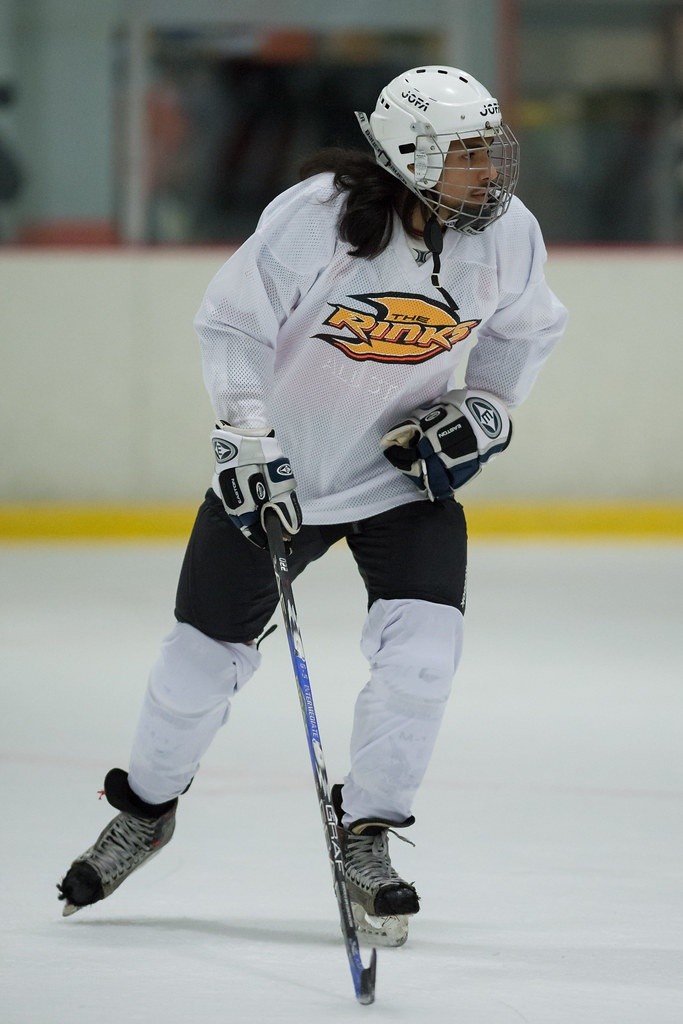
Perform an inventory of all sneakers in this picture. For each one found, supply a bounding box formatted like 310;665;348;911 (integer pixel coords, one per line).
57;768;186;916
323;785;420;947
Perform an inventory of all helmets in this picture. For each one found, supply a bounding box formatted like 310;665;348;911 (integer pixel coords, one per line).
366;66;504;191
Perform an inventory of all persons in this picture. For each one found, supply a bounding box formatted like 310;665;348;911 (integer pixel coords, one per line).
56;66;567;947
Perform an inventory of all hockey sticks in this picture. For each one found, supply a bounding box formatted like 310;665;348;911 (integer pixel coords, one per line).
262;506;379;1007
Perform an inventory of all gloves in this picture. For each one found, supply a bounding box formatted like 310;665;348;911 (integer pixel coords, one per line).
380;391;511;501
210;425;304;559
